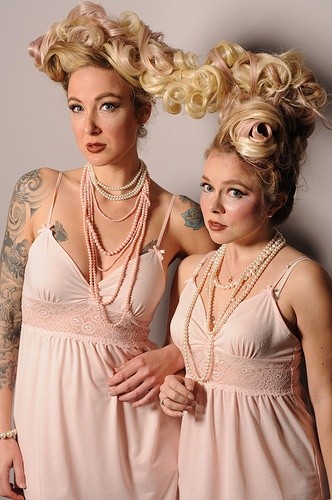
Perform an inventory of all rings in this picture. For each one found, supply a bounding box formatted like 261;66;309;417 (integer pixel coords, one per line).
161;397;170;406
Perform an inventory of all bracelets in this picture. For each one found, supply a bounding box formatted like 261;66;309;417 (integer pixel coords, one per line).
0;429;17;439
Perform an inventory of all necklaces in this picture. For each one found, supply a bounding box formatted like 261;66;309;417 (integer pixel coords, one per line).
88;159;147;201
225;248;257;282
80;158;152;326
181;228;288;383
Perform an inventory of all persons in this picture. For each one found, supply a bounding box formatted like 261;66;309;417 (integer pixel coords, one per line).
0;0;221;500
159;40;332;500
